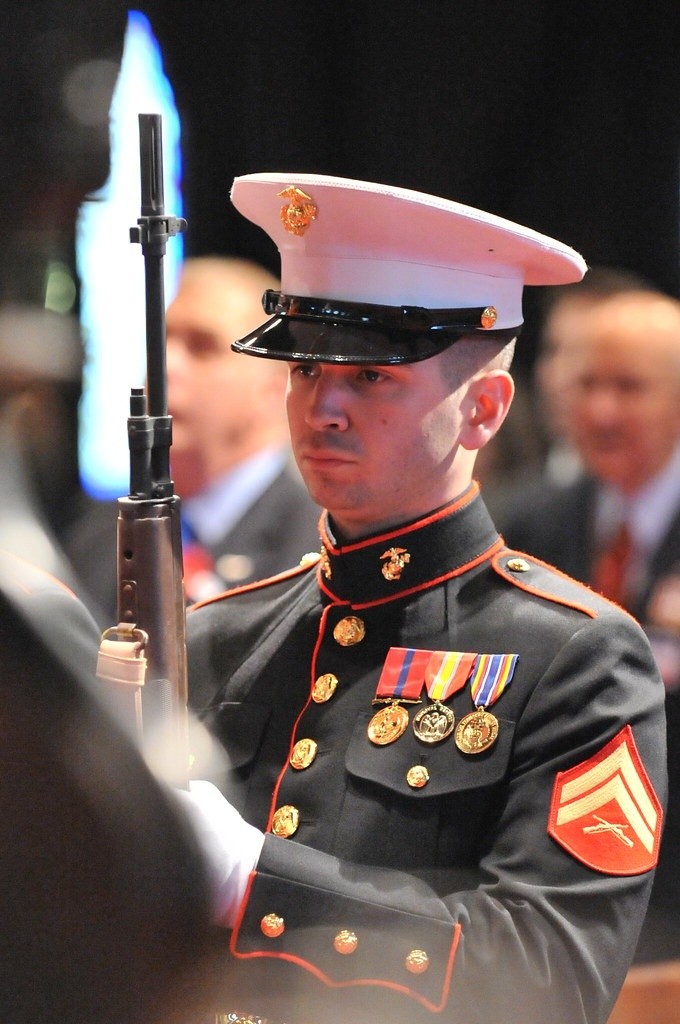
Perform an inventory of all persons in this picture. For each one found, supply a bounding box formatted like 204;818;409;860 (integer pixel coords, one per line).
111;256;318;609
500;286;677;647
165;173;666;1016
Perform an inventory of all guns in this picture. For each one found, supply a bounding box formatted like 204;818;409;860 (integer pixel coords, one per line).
112;108;190;718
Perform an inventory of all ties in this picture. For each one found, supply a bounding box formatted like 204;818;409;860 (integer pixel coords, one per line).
174;514;213;598
591;518;633;605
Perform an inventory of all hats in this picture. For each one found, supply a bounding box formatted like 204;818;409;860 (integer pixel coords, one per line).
230;171;586;367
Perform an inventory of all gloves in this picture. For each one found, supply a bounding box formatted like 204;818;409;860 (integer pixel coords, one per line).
166;777;264;928
